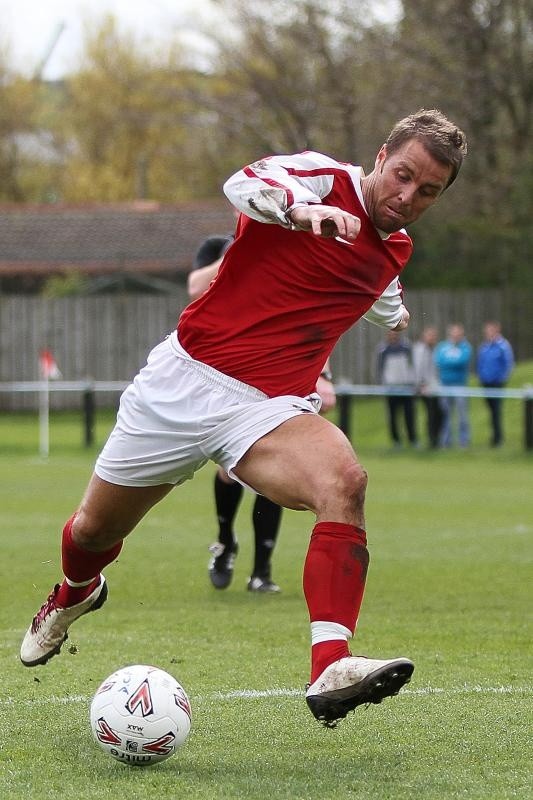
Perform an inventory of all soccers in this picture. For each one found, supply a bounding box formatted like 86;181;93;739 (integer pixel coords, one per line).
87;665;195;771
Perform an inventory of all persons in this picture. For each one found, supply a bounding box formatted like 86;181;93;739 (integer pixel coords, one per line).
17;105;468;726
371;328;420;454
185;209;339;596
471;319;514;450
410;327;449;452
432;322;474;451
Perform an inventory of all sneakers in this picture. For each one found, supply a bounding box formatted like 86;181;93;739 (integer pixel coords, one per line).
246;574;281;594
306;656;416;723
19;573;108;668
208;535;240;589
